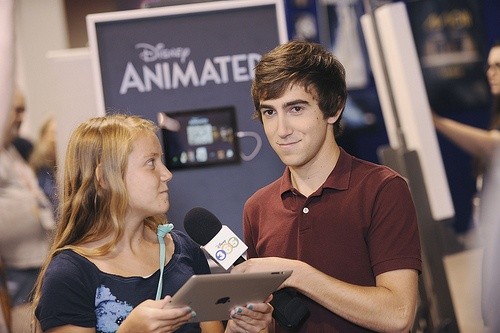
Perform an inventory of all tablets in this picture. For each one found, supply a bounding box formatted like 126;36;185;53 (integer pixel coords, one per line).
160;269;294;323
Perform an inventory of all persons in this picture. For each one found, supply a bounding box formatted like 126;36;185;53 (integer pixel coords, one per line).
231;40;422;333
433;40;500;333
28;115;274;333
0;87;59;333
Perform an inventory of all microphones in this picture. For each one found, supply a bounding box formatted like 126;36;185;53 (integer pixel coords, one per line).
183;206;247;269
